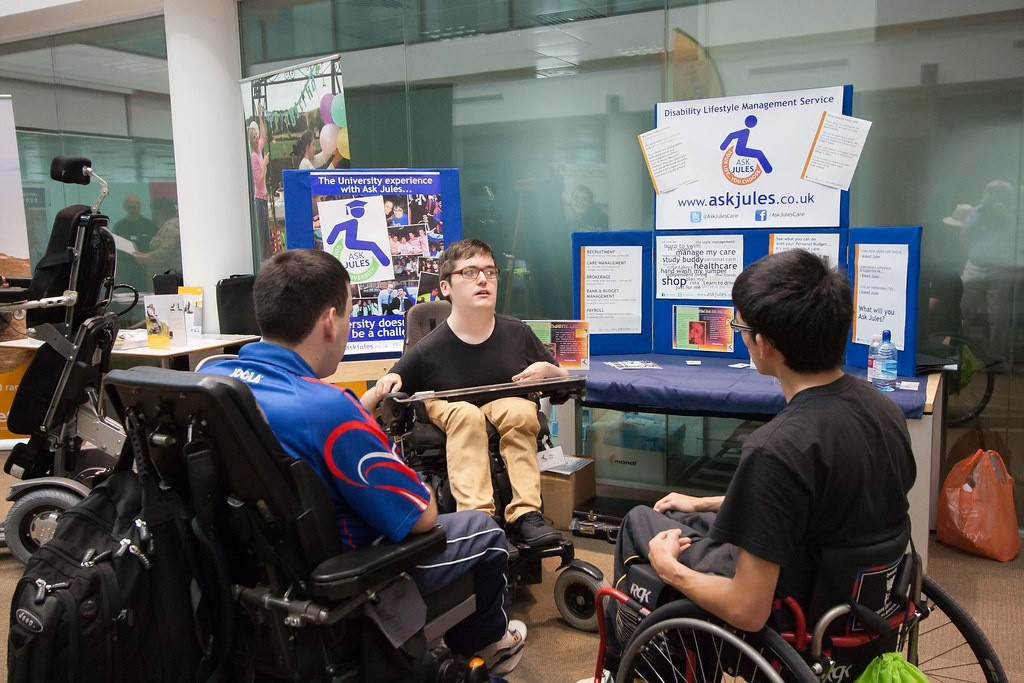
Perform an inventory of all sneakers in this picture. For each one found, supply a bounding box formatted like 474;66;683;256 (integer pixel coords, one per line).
506;510;562;546
505;540;519;561
454;619;527;672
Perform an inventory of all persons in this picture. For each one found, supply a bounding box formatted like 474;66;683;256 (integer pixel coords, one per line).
313;194;443;318
918;218;964;358
110;196;161;292
566;181;610;232
249;103;270;262
690;323;704;344
375;239;567;546
944;203;977;239
195;249;528;677
576;249;917;683
465;182;503;313
134;197;182;294
291;131;345;171
962;180;1024;363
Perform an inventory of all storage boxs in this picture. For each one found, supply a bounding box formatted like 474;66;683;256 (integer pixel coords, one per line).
541;455;596;531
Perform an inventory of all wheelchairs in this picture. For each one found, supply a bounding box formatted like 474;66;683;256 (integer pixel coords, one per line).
920;272;1006;428
378;300;610;633
105;367;512;683
593;513;1009;683
0;156;139;561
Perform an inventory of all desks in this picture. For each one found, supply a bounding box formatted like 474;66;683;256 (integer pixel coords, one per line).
0;329;262;369
556;352;946;602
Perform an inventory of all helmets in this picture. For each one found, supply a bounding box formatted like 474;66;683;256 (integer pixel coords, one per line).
930;270;963;304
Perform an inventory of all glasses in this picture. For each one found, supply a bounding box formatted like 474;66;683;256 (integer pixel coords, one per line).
444;266;500;280
730;318;773;347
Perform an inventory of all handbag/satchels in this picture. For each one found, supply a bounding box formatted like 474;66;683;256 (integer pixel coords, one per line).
933;448;1022;563
961;345;979;385
153;270;184;295
848;601;931;683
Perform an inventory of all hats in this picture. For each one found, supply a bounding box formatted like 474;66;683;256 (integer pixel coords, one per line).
943;204;972;227
394;285;405;289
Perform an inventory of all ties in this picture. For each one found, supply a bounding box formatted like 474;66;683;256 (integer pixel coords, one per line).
400;297;405;312
388;295;390;307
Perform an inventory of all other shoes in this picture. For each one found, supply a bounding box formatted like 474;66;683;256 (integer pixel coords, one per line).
577;670;615;683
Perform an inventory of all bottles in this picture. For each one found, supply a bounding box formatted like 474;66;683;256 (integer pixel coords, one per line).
873;330;897;393
866;335;882;382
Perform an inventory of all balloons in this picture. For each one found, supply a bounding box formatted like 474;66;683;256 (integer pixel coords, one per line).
320;92;352;160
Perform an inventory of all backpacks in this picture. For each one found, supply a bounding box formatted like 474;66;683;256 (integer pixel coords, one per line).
7;418;232;683
